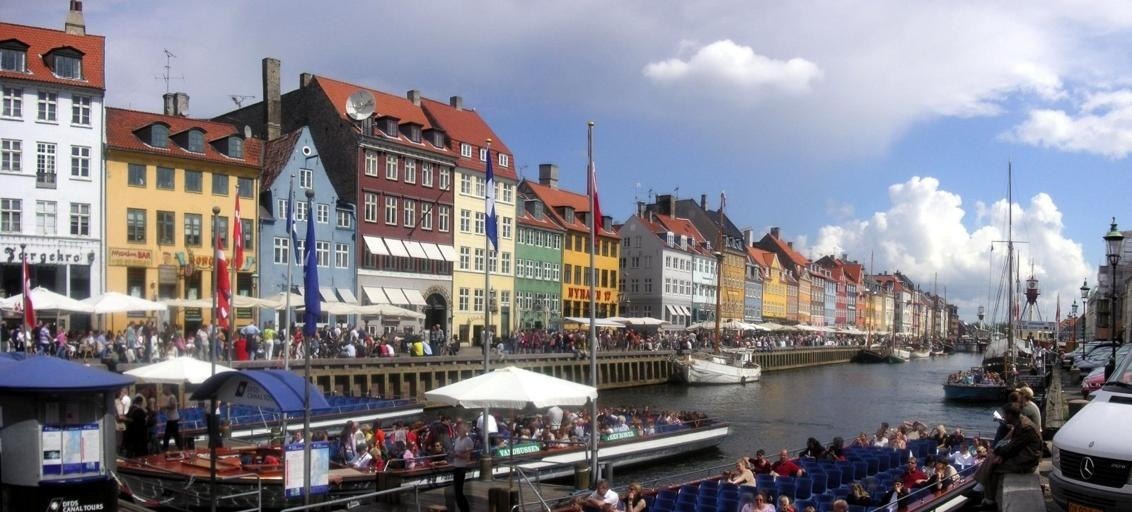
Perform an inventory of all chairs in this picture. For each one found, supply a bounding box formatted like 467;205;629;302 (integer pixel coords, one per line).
630;437;941;512
150;392;410;437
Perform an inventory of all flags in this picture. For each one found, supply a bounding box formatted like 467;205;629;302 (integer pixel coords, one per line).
22;254;36;329
233;191;245;271
285;181;297;265
486;150;498;255
217;236;231;333
303;199;320;337
586;155;601;245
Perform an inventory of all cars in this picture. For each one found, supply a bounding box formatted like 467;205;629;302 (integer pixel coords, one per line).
1055;339;1132;399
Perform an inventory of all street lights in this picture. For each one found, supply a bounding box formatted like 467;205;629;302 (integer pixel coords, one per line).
1080;277;1091;360
1066;311;1072;340
1099;217;1124;369
1072;299;1078;350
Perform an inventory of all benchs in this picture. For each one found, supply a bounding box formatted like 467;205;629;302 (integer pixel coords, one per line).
379;352;401;368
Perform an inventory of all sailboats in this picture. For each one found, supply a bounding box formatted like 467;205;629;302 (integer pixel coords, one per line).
672;190;762;386
856;251;990;363
941;159;1052;404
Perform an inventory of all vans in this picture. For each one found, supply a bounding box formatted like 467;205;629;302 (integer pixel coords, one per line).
1049;342;1132;512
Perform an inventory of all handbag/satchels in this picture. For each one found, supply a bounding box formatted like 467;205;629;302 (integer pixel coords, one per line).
1042;442;1053;458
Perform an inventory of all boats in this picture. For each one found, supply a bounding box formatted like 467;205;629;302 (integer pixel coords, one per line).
514;423;1001;512
117;391;426;451
116;405;732;508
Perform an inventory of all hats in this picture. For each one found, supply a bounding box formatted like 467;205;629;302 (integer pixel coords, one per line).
1015;386;1034;397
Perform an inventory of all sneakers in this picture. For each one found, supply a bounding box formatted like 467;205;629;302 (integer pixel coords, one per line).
960;486;984;500
